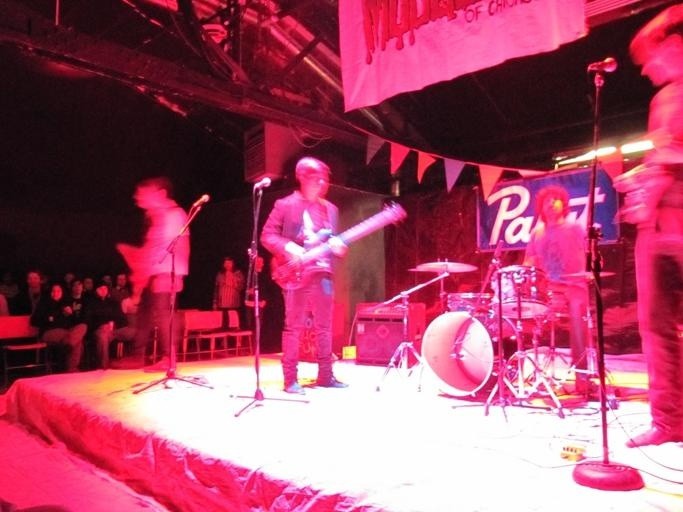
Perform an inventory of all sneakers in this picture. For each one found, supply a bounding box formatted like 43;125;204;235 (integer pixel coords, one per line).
318;376;347;388
625;427;682;448
143;357;177;372
286;381;307;395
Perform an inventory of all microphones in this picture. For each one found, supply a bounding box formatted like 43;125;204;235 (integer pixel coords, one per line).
193;194;210;206
585;56;617;74
255;177;271;188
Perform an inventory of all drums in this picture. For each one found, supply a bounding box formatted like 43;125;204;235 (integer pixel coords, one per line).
486;263;553;320
420;310;525;398
444;292;504;342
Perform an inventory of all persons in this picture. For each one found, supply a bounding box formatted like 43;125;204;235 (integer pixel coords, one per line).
521;183;596;398
259;156;353;397
14;256;276;377
608;0;682;448
116;173;195;378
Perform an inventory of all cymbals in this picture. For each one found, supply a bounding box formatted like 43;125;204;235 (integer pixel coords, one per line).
557;269;618;283
416;261;478;276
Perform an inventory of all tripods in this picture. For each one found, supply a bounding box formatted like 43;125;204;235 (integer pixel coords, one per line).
484;275;618;421
133;248;214;394
229;261;309;418
376;308;422;392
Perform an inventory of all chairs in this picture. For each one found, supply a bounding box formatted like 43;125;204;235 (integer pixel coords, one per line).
172;308;254;365
1;316;49;382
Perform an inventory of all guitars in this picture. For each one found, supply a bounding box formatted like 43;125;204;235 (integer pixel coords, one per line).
269;200;410;294
